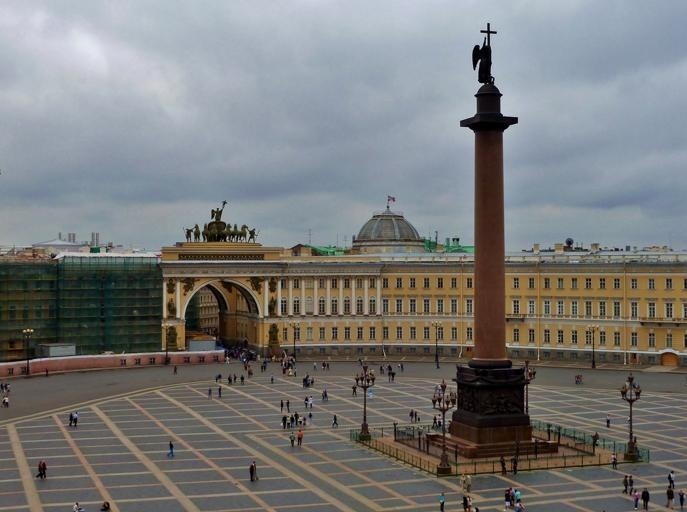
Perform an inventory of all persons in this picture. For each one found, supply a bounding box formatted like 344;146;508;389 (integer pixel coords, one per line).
0;380;11;406
208;341;329;446
525;366;538;380
73;410;78;428
167;439;174;457
248;228;258;243
69;411;73;425
634;436;641;457
173;364;177;375
595;432;599;440
409;383;452;428
351;361;403;395
74;501;110;511
607;413;611;427
250;461;259;483
35;460;47;479
212;208;224;220
440;473;522;511
611;455;686;510
332;415;338;426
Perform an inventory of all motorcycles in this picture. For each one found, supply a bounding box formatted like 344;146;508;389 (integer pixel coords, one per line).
242;357;249;364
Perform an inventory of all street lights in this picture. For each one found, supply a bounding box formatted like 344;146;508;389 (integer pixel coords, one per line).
287;320;303;360
430;320;443;360
618;372;640;462
520;359;536;417
21;328;34;375
429;378;457;474
356;363;374;443
587;324;600;367
159;323;173;364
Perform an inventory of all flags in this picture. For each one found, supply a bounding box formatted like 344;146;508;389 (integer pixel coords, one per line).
389;196;397;205
